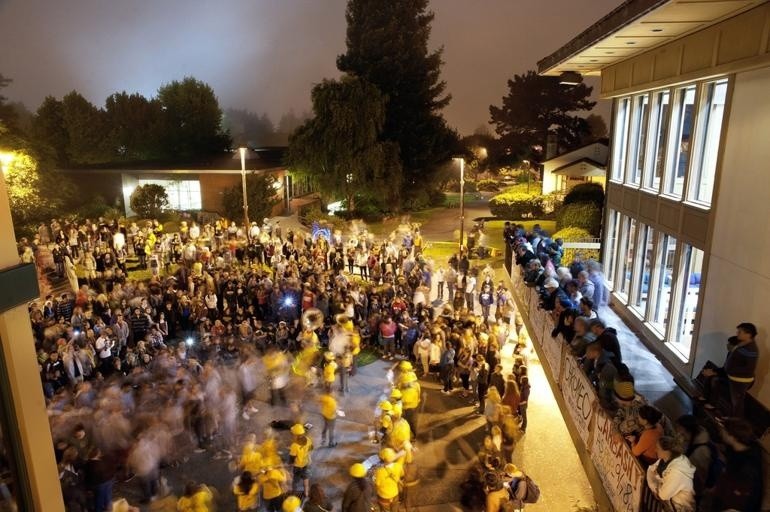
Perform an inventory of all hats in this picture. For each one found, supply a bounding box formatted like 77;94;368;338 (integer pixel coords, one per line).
503;463;520;476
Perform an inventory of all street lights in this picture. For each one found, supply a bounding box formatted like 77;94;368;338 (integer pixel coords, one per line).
451;157;464;257
238;147;249;243
523;160;530;194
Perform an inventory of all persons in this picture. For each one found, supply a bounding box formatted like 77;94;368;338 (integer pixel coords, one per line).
500;223;770;511
1;210;539;511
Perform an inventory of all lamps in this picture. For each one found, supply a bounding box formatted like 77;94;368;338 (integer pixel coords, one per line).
559;72;583;86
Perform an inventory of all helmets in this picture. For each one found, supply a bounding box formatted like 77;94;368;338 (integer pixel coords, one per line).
281;350;417;512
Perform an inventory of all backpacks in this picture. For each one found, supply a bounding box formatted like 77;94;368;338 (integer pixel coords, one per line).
515;474;540;504
